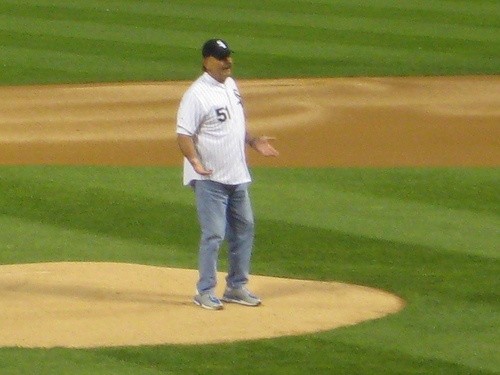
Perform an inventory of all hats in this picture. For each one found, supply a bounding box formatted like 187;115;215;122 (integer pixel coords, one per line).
202;38;233;60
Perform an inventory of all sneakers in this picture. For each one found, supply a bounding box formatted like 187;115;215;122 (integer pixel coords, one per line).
221;286;263;306
194;287;223;311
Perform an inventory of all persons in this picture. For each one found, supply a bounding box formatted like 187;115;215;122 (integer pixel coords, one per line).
175;39;280;310
